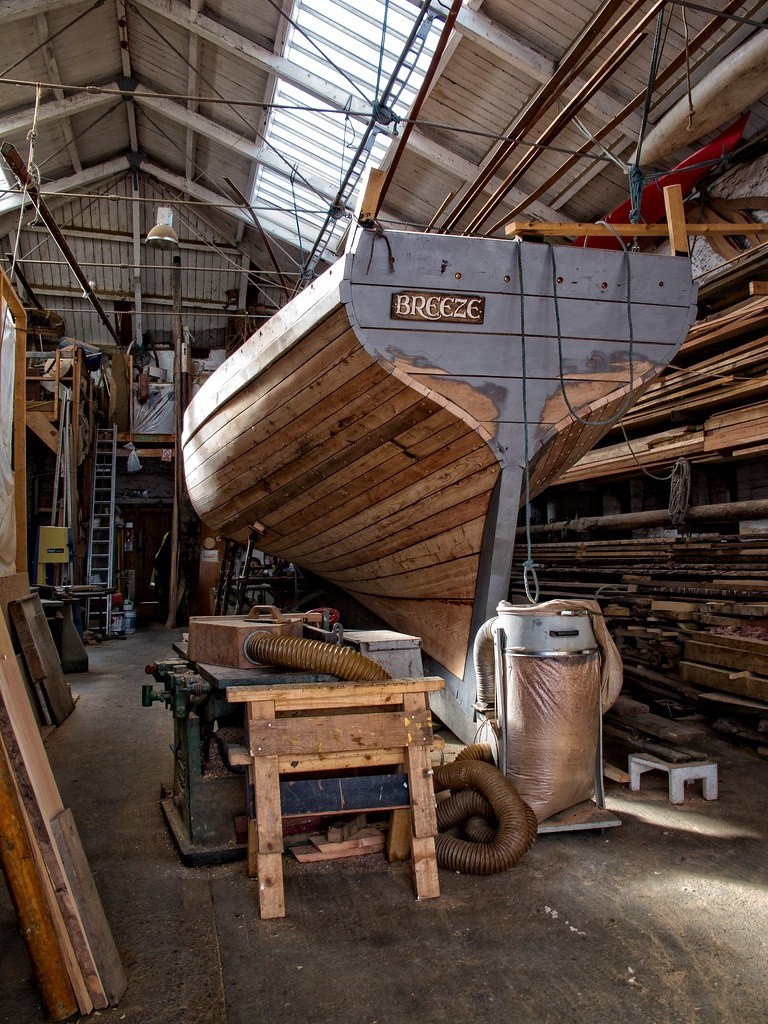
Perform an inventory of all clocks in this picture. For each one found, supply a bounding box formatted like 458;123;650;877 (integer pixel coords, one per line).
202;536;217;550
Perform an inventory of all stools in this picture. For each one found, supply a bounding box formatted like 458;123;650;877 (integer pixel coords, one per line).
628;753;718;805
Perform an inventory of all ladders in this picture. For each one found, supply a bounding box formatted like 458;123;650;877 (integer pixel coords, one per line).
85;423;117;635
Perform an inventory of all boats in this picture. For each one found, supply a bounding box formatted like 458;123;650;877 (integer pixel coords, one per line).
181;165;701;680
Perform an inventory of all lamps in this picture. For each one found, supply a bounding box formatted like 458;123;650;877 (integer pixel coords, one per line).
145;206;179;250
